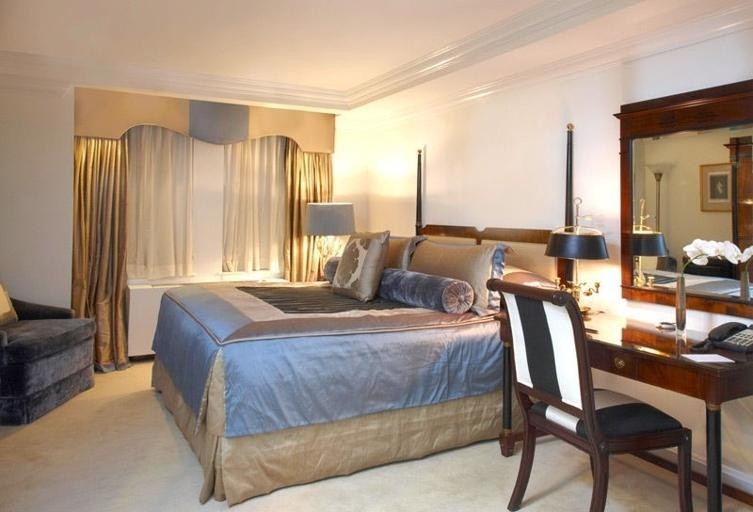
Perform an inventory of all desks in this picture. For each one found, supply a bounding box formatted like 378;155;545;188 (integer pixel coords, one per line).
493;310;753;512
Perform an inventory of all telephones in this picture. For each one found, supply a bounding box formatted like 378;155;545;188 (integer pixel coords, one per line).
692;323;753;352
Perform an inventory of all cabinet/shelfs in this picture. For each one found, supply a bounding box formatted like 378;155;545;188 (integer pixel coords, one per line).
723;136;752;280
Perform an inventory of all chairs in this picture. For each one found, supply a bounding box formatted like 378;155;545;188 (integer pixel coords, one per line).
485;279;692;511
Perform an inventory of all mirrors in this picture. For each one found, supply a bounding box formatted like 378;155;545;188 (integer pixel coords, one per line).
613;79;753;319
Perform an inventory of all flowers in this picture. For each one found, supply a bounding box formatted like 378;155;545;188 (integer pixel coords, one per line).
680;238;717;279
721;241;752;270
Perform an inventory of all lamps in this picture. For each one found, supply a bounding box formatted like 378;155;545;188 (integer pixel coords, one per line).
544;196;610;334
304;202;355;280
633;199;670;289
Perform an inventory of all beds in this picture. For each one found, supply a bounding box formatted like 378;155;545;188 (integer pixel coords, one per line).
151;224;574;509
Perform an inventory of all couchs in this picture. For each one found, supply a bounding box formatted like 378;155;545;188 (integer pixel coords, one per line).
0;280;97;426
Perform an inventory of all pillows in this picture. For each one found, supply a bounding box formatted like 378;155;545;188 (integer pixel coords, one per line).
325;258;475;314
386;236;427;268
331;230;390;303
406;238;518;318
0;280;18;328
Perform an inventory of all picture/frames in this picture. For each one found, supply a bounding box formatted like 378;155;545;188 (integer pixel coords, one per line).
699;163;733;212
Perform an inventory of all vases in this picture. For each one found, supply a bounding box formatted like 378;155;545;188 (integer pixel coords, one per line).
675;277;686;361
739;272;750;305
